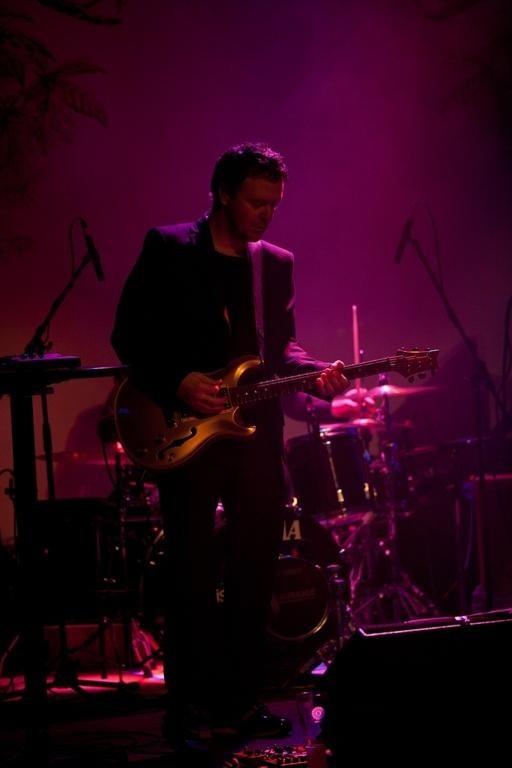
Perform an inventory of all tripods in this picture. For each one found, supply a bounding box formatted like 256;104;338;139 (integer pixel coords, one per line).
10;274;137;699
287;435;437;685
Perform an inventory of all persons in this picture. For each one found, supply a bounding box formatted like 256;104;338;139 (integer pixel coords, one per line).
111;143;351;748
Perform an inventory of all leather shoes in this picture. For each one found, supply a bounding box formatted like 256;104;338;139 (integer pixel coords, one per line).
235;704;296;740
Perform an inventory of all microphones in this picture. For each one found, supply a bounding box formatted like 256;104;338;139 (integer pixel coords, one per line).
393;217;414;264
79;222;105;283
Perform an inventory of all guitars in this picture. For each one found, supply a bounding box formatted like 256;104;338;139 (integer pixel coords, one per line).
112;347;439;467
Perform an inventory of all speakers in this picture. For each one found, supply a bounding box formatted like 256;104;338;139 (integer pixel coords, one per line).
311;607;512;768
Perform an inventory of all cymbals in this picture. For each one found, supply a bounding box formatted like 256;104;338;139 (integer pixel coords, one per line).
368;382;438;397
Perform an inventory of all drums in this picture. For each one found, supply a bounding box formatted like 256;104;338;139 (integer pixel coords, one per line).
135;503;345;691
287;425;366;512
